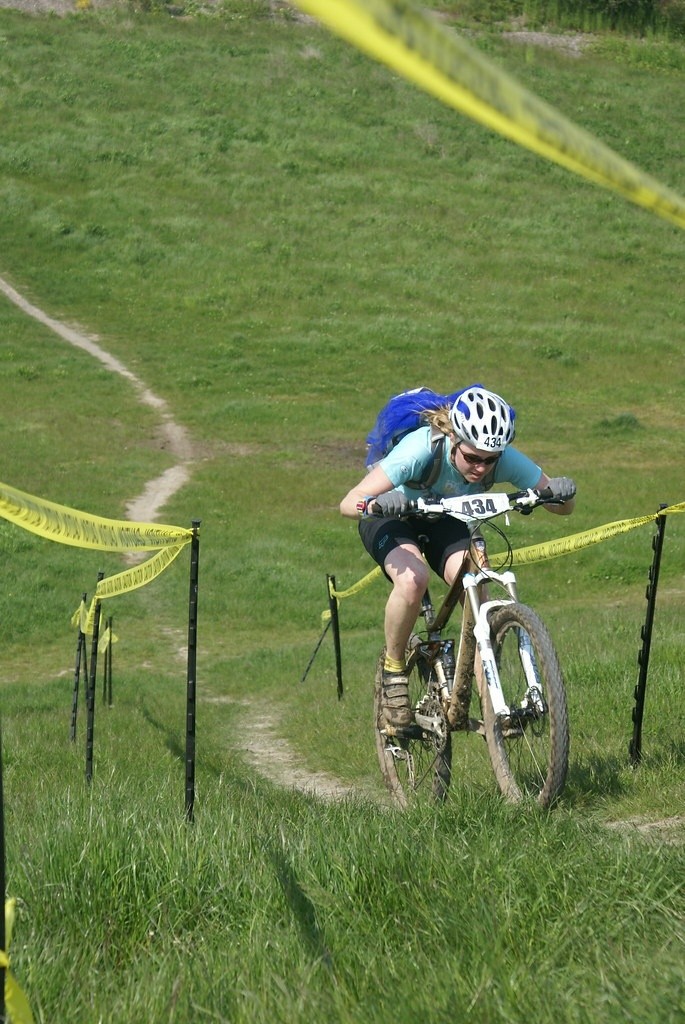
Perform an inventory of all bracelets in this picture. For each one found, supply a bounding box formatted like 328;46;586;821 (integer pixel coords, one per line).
356;496;378;522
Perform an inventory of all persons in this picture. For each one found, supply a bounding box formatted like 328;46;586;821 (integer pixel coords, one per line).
339;387;577;737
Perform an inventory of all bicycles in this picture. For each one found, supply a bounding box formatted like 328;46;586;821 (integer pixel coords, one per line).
370;487;571;818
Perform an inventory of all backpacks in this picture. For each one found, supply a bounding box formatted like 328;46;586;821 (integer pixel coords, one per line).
366;383;497;491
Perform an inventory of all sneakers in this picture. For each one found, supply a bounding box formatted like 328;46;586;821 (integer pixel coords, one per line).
378;646;413;727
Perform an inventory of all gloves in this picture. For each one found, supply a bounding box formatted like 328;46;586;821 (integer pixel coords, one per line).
544;476;576;506
370;490;410;520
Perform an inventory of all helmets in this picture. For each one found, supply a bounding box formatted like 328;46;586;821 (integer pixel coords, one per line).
450;386;515;453
